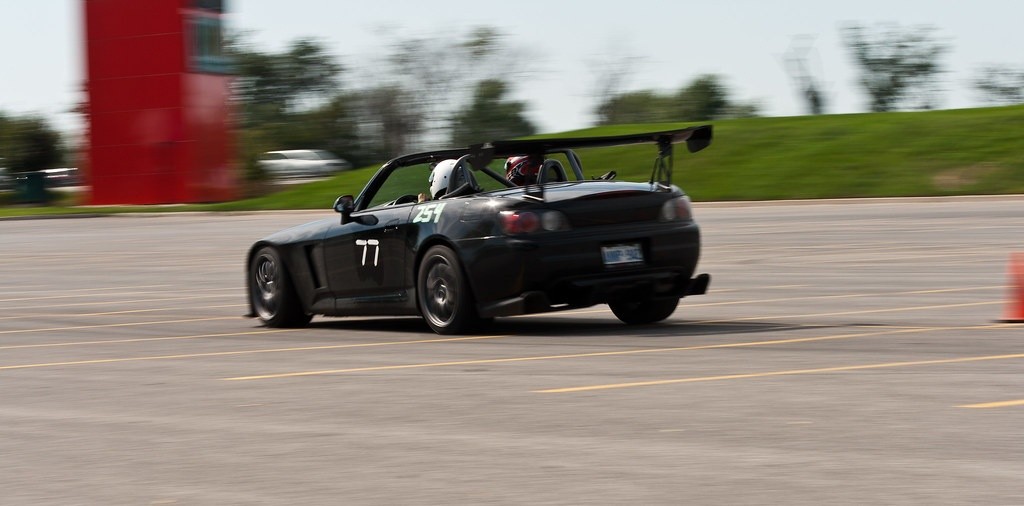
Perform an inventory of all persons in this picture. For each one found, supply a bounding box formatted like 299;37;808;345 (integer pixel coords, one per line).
418;158;468;202
504;153;546;189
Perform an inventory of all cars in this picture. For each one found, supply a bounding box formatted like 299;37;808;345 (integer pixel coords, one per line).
242;125;715;336
41;168;80;188
0;168;21;193
255;149;350;179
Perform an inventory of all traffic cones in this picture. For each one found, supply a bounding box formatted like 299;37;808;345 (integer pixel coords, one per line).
989;252;1024;323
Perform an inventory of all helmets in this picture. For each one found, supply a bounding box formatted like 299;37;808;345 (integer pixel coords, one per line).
429;158;477;200
504;156;542;184
